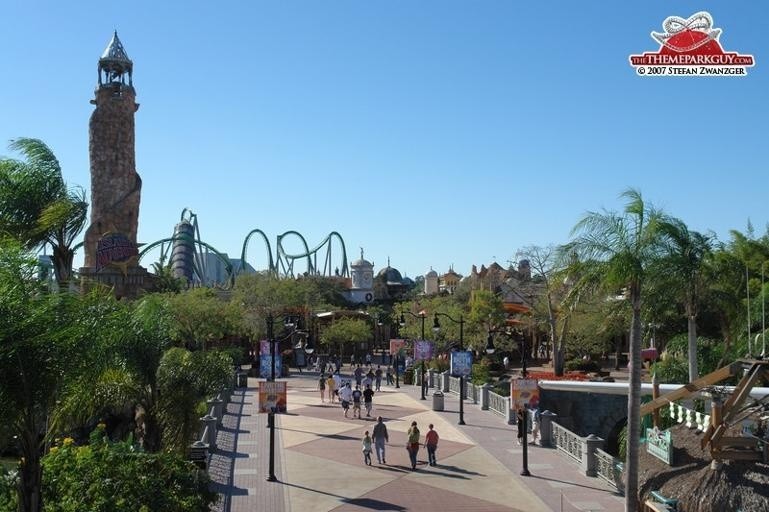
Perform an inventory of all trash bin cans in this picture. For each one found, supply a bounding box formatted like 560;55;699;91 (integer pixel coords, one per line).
282;364;289;376
239;373;247;387
432;390;444;411
404;367;414;384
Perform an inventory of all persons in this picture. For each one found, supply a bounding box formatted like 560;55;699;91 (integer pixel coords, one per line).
423;424;440;467
305;344;431;422
361;430;373;467
372;415;389;465
503;354;510;370
406;425;420;471
515;406;525;446
538;343;546;361
408;421;420;435
527;405;541;446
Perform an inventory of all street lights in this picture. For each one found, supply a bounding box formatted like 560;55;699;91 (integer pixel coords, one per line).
266;326;314;482
487;329;531;477
433;311;466;426
378;312;400;389
400;311;428;400
265;312;294;381
